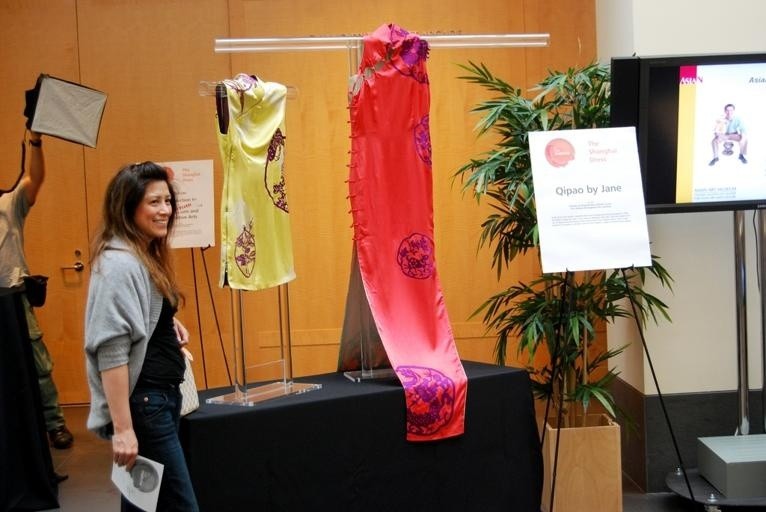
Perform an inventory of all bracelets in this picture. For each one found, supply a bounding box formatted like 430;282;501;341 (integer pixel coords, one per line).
29;139;41;146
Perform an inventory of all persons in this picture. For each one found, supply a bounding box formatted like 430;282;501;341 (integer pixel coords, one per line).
84;162;200;511
0;133;73;448
707;103;746;167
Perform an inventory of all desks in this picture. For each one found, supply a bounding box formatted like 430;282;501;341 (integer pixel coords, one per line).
179;359;545;511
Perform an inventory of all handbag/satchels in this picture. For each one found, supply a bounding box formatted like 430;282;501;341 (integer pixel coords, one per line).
176;346;200;419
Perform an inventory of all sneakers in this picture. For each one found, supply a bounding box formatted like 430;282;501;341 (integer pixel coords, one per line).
738;153;747;164
709;158;719;165
49;425;73;449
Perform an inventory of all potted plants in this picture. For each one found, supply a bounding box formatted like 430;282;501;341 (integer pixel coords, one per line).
448;53;672;512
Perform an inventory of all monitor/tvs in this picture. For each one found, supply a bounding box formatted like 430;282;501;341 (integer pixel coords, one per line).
609;53;766;221
